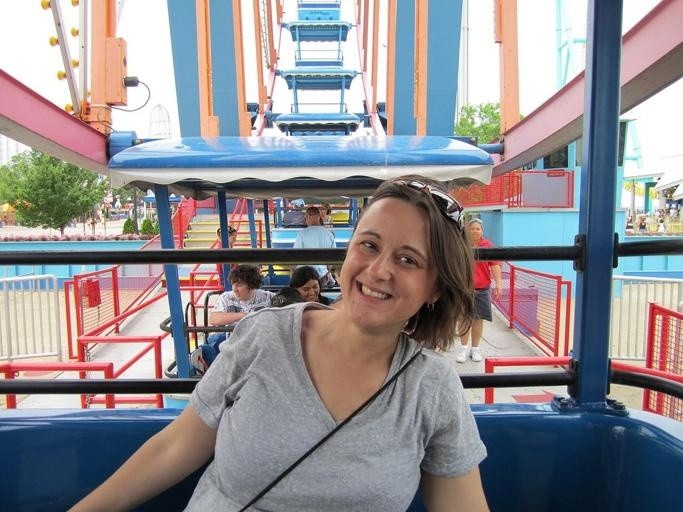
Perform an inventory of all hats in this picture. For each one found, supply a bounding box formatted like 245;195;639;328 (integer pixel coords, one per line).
291;200;304;207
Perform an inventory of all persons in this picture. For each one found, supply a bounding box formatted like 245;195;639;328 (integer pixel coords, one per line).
452;216;503;364
197;199;344;369
64;174;491;512
625;202;680;234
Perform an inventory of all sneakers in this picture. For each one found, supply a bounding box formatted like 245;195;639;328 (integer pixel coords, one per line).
457;345;467;361
470;347;481;361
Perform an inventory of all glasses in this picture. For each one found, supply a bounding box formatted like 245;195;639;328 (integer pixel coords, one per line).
392;179;465;235
